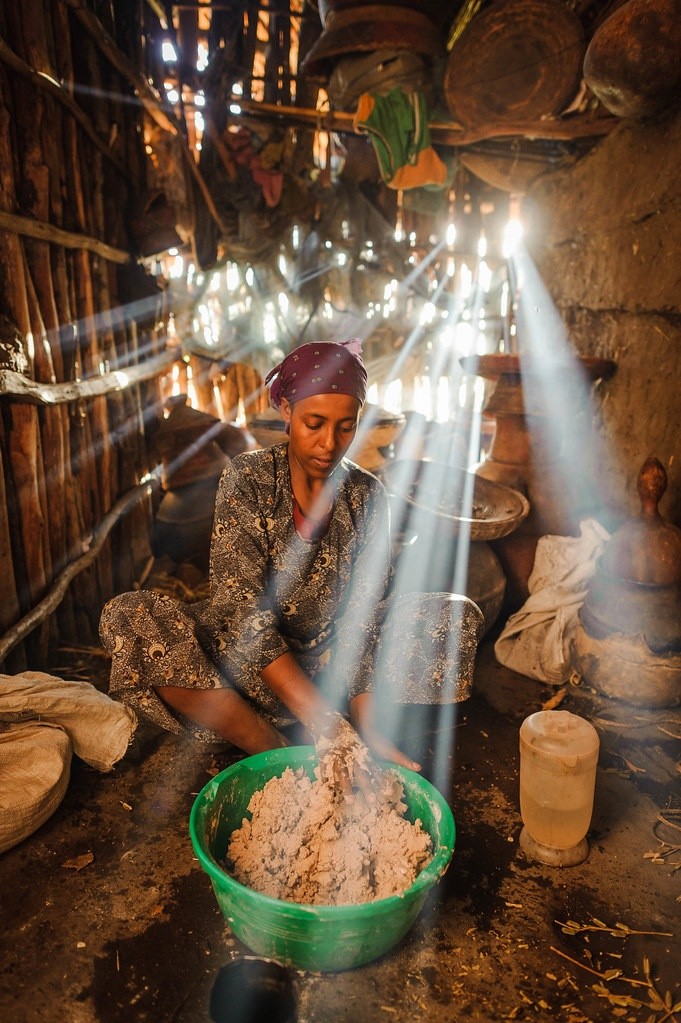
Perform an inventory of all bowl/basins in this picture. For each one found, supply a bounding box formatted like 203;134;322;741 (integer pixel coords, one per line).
189;744;456;973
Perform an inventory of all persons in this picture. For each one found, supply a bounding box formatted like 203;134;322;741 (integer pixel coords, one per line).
98;339;486;806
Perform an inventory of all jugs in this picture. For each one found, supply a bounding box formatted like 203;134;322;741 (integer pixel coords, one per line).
520;712;599;850
